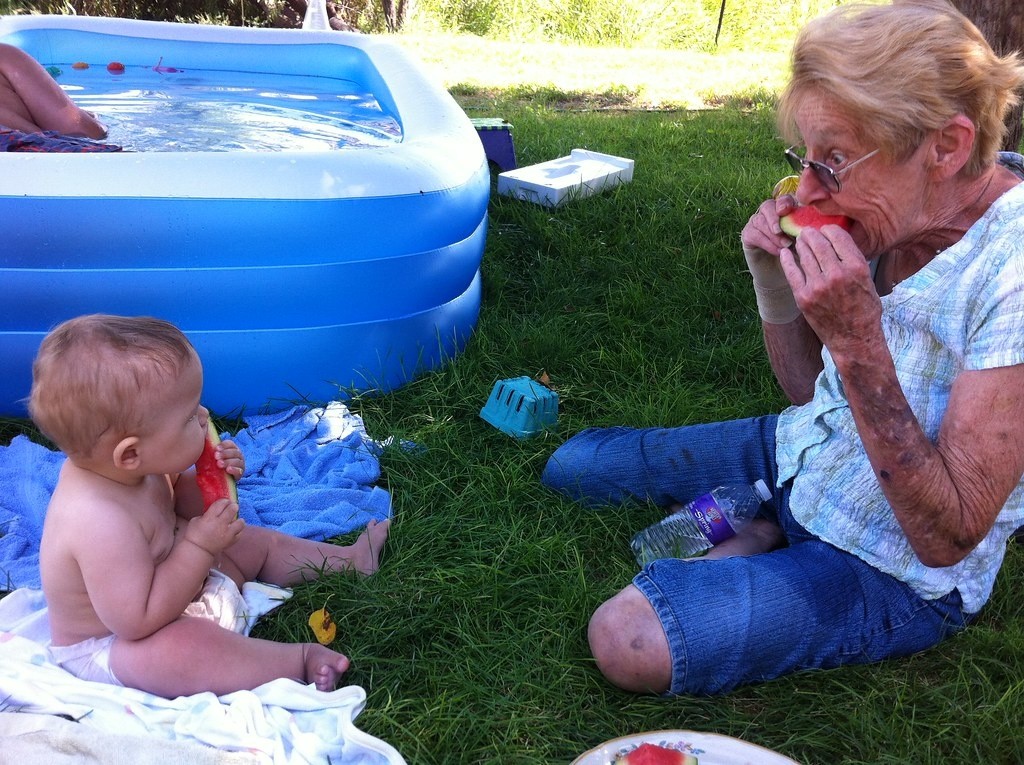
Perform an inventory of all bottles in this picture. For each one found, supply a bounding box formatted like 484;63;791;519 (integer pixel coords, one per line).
631;476;772;569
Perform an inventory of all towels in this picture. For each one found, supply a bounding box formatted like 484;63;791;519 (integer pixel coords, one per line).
0;401;408;764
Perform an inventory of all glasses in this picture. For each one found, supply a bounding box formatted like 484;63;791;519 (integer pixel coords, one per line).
784;140;881;194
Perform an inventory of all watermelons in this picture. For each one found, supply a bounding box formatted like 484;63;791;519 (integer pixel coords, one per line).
614;744;698;765
780;204;852;240
196;416;239;521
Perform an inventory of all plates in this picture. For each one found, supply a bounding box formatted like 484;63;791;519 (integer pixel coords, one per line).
569;730;800;765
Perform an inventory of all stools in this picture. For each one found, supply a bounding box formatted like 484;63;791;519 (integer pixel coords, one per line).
470;118;515;172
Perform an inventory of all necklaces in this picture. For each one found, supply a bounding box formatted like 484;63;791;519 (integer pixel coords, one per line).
890;168;998;291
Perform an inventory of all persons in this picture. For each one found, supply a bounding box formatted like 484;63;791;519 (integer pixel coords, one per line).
0;40;109;141
542;0;1023;698
26;311;391;701
